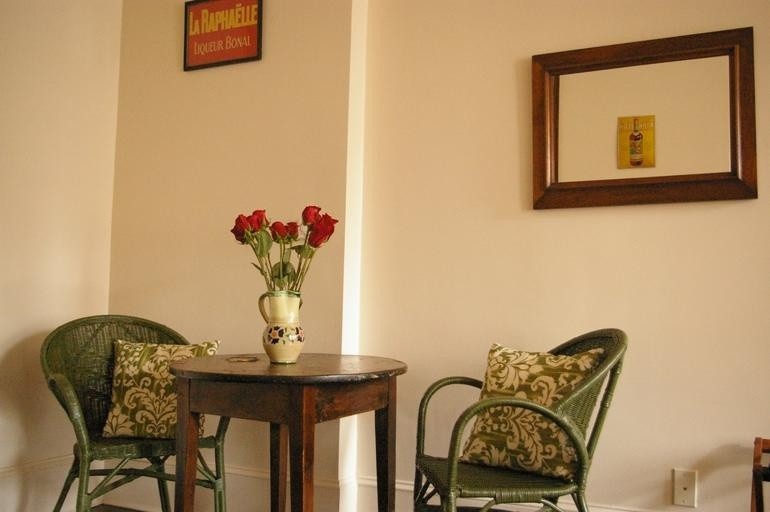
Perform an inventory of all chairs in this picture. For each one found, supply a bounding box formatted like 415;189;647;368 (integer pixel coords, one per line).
413;328;629;511
41;315;231;512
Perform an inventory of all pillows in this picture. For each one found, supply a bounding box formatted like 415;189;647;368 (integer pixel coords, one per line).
102;337;223;440
458;341;605;481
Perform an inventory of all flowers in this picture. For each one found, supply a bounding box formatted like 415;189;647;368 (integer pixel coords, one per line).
226;201;341;288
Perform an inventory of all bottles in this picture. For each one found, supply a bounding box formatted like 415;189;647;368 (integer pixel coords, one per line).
628;115;643;166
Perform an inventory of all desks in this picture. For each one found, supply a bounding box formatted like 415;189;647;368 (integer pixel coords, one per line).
165;351;408;512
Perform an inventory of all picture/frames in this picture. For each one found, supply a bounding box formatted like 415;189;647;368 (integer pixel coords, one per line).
179;1;264;70
524;26;757;214
